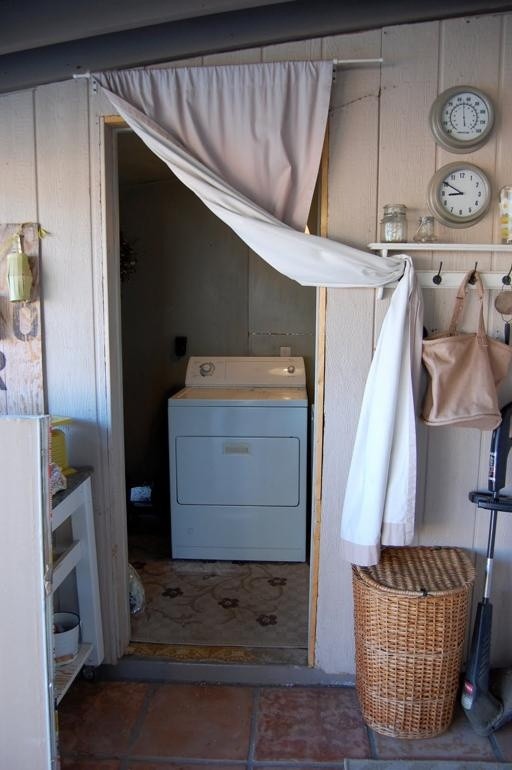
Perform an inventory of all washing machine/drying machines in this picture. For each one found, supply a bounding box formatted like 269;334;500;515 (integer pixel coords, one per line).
167;356;307;564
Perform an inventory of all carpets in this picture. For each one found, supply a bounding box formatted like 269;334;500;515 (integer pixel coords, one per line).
130;557;309;649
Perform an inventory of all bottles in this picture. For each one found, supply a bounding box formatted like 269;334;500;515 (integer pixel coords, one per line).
413;214;436;243
498;185;512;245
380;202;408;243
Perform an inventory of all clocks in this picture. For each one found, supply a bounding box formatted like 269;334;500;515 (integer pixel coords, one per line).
426;162;492;224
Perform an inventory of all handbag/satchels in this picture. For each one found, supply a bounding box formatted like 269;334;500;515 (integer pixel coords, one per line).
416;268;512;432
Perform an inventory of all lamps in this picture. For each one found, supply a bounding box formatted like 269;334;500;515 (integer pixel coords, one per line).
7;234;34;302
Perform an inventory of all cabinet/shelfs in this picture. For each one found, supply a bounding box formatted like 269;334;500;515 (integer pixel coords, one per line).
47;466;129;710
